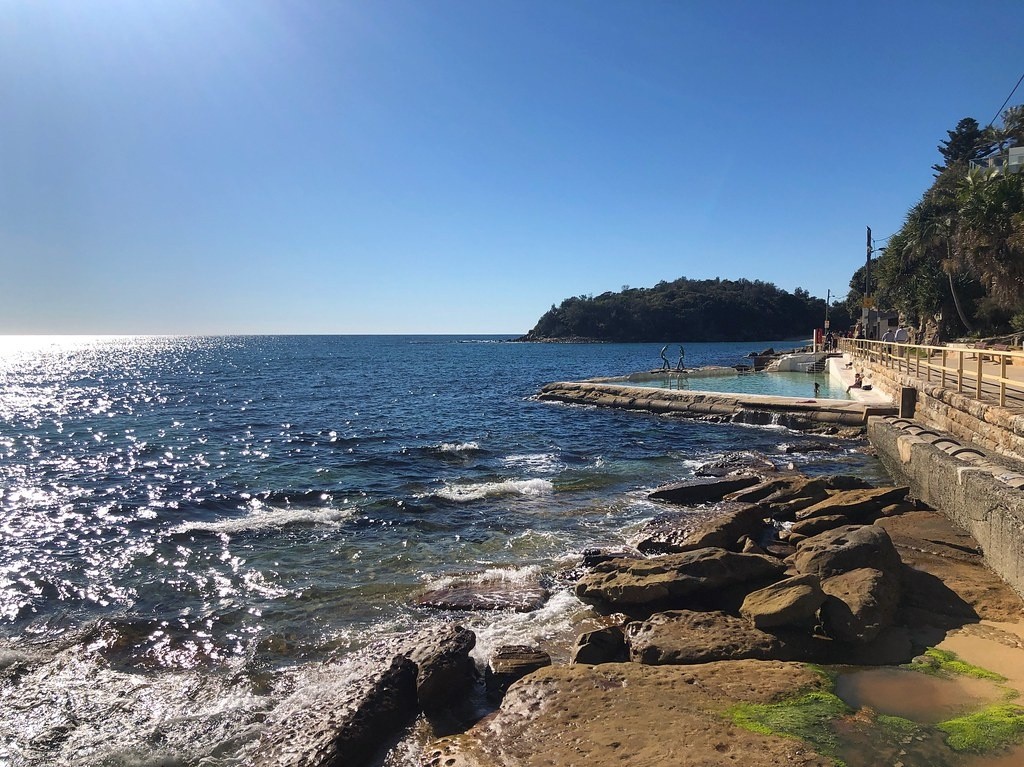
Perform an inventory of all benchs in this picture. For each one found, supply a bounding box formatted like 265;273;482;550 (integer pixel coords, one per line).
972;342;1014;365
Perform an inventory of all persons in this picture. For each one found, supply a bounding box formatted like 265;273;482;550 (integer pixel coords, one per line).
894;324;908;357
882;328;894;361
846;373;862;393
